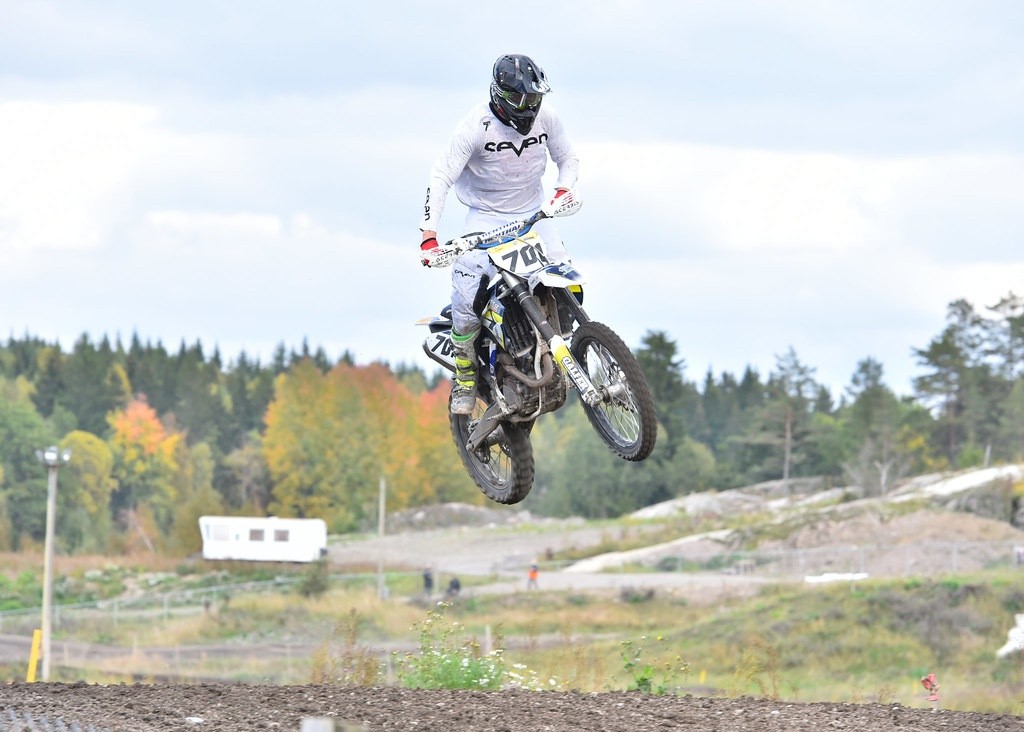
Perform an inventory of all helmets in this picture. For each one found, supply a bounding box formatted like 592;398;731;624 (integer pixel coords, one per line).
489;54;554;136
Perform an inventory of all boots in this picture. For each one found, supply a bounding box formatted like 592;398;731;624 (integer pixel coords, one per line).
450;328;482;414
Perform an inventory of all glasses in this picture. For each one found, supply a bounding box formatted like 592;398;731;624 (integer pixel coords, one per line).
492;81;543;110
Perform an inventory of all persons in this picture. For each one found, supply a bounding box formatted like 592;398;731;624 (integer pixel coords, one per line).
418;53;584;414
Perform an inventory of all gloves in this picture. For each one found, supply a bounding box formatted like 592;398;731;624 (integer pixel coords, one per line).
550;190;583;217
420;237;454;268
